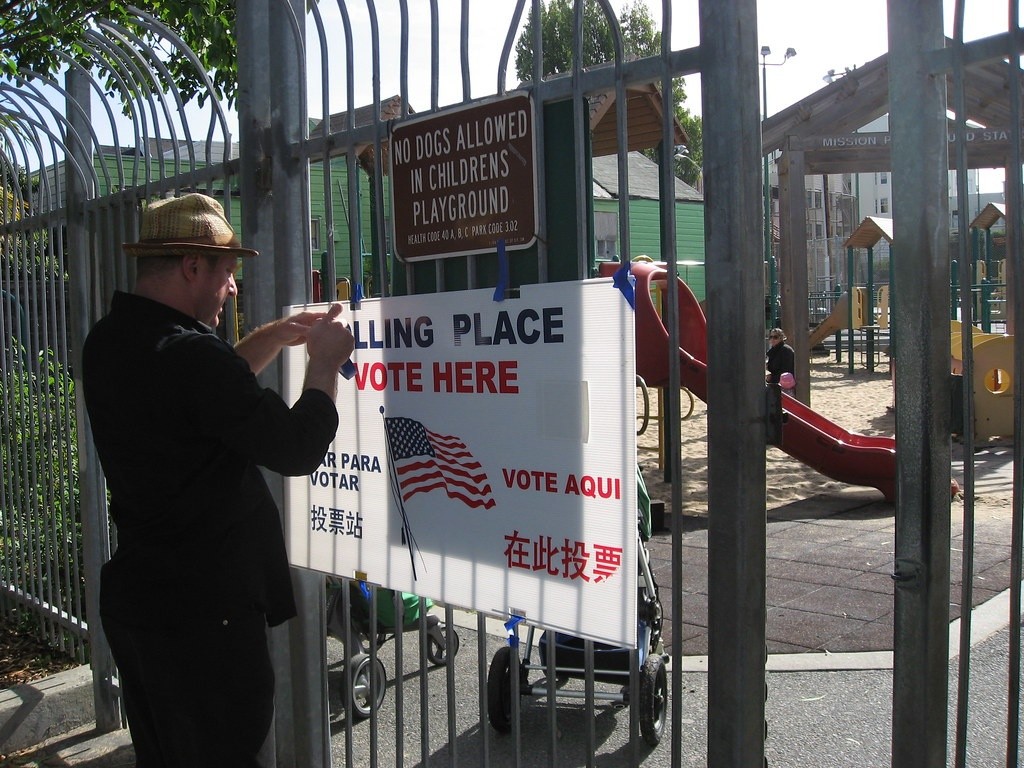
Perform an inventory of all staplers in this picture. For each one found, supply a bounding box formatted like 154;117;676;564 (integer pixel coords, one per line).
339;360;358;382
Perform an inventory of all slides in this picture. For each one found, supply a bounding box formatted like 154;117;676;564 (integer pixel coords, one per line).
808;290;847;350
624;265;961;505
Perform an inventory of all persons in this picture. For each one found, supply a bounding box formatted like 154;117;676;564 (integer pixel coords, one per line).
82;193;355;768
765;328;795;397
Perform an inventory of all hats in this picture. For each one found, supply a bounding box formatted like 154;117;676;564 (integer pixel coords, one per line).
779;372;796;389
122;192;259;258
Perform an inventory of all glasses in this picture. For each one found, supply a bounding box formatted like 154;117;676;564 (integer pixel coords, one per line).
769;334;781;340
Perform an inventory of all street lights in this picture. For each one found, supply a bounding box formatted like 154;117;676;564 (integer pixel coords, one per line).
759;45;799;326
823;66;859;373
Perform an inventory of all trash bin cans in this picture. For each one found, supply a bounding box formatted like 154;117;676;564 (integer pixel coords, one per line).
809;322;824;351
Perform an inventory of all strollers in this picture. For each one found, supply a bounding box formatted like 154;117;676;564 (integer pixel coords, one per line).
321;576;459;718
486;453;670;747
